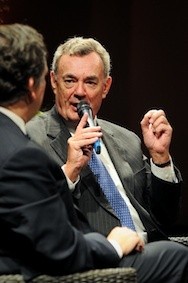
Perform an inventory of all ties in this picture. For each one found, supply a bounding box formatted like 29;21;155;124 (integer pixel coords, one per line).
88;149;136;233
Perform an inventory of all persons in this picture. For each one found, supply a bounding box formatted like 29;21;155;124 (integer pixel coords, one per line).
0;23;188;283
26;38;188;248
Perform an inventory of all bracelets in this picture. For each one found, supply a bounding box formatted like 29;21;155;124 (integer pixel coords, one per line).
154;160;170;168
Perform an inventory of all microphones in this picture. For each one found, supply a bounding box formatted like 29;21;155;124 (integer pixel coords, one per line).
77;101;101;155
